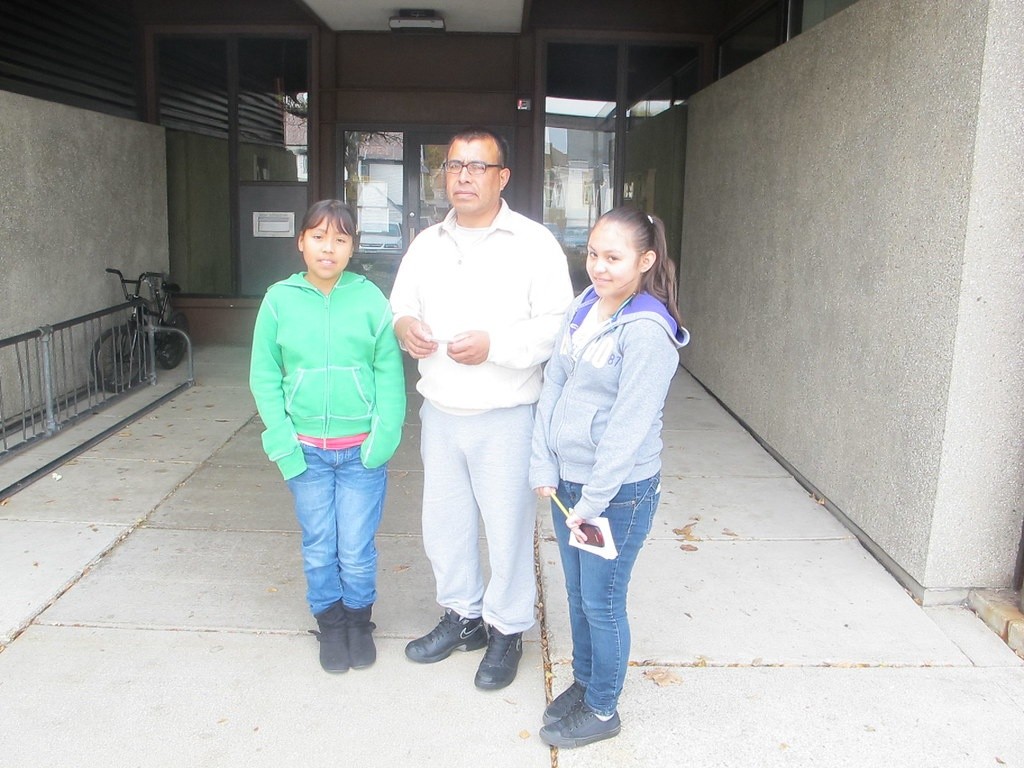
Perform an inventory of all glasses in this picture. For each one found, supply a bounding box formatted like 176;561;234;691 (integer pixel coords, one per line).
443;160;503;174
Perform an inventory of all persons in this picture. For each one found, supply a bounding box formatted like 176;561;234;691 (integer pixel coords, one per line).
527;203;690;747
248;199;407;672
390;129;576;692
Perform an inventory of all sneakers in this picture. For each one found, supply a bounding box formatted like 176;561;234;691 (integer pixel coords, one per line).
405;607;487;663
475;622;523;690
543;681;586;724
540;704;622;747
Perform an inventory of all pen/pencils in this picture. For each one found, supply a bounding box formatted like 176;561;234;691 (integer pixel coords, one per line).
549;490;570;519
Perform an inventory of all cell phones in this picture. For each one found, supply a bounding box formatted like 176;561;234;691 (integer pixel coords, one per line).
577;523;604;547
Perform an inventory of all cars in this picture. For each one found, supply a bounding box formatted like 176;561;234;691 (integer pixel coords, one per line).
356;222;404;255
545;222;597;248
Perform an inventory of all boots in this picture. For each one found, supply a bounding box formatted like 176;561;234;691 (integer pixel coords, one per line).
311;600;376;676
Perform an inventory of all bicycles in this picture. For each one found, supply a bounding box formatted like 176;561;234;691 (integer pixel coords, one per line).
90;267;191;392
